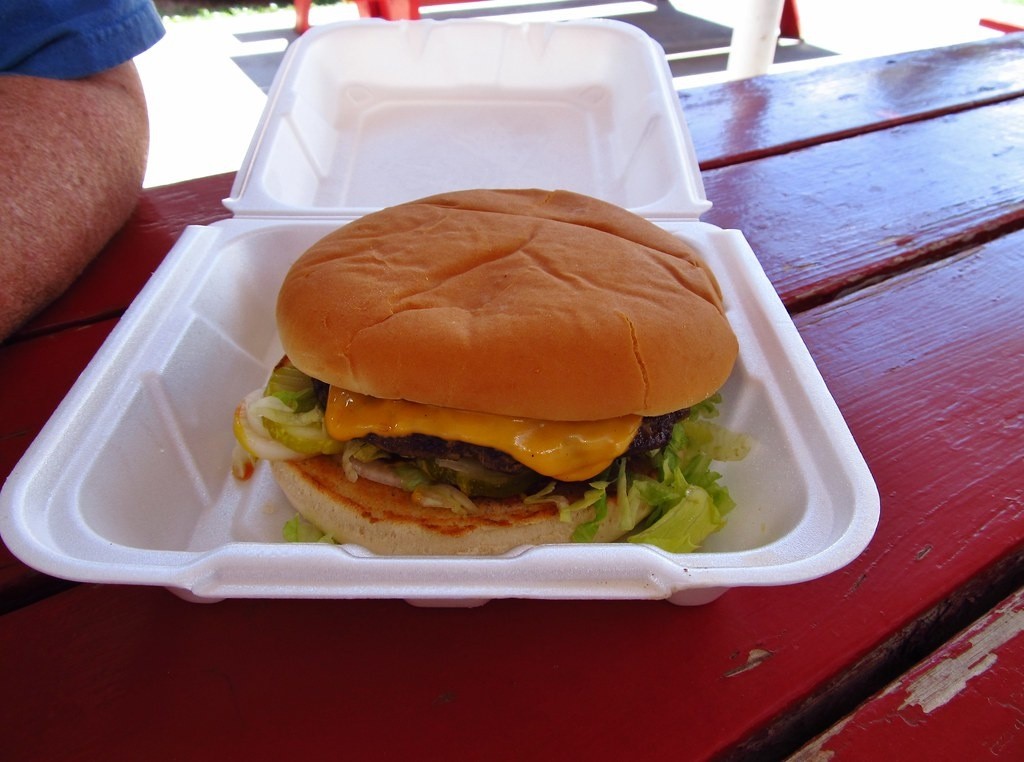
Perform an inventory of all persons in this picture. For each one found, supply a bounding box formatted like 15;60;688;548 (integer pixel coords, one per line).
0;0;149;349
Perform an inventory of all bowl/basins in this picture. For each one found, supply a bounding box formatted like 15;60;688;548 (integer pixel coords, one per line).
0;17;882;605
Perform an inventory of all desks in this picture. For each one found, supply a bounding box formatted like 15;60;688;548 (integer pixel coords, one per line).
0;29;1024;762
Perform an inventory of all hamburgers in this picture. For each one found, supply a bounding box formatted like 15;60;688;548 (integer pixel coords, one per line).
230;189;754;557
